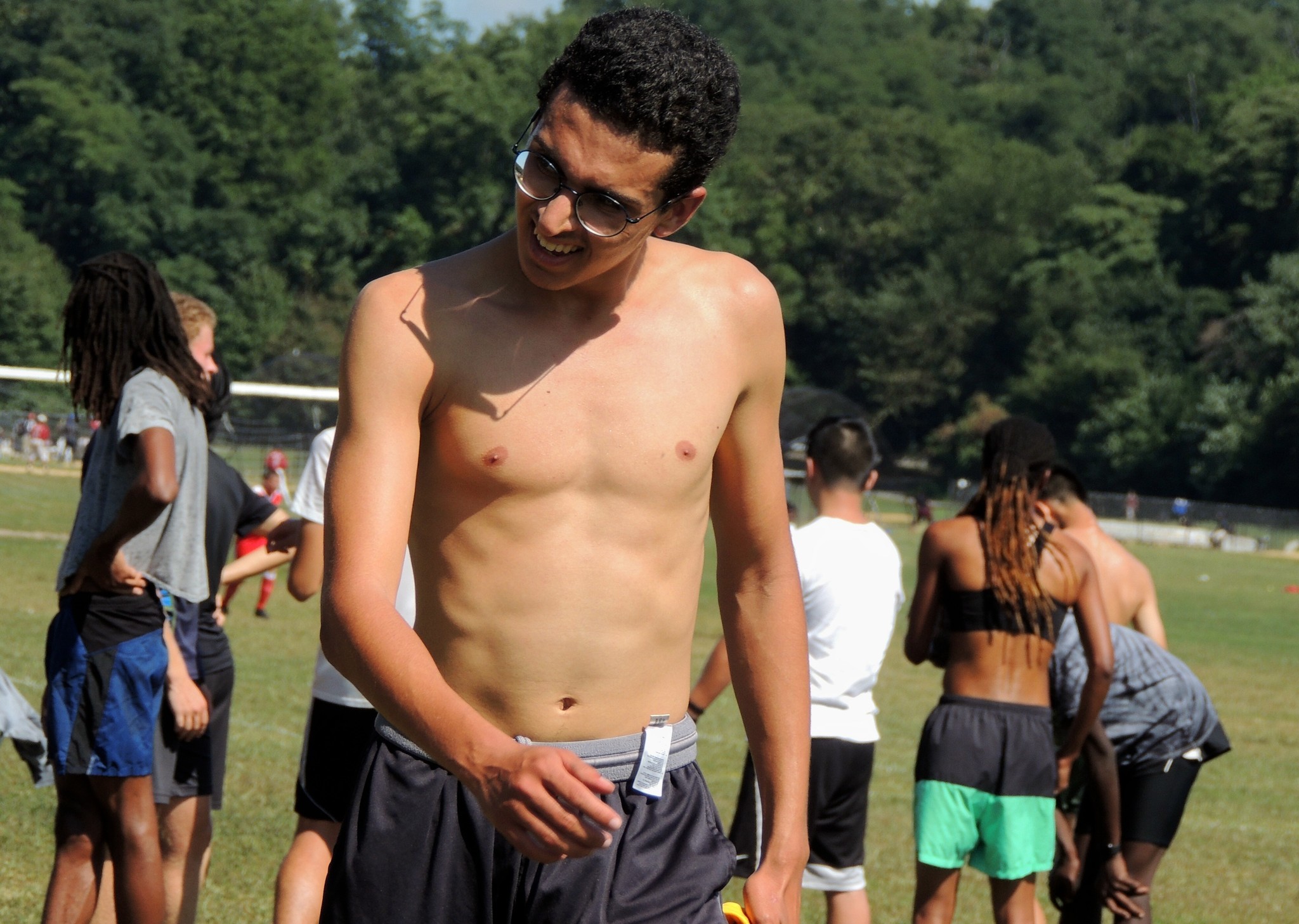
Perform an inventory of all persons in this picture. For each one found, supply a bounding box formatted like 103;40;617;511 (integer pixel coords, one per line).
1124;486;1138;520
273;426;416;924
1046;608;1232;924
1036;459;1165;822
689;413;907;924
906;419;1116;924
320;7;813;924
908;479;934;527
1171;497;1188;525
38;237;295;924
10;412;81;464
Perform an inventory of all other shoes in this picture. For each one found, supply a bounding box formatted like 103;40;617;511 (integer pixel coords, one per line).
254;610;268;619
220;606;229;614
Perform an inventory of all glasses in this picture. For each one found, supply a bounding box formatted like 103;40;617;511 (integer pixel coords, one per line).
512;105;692;239
